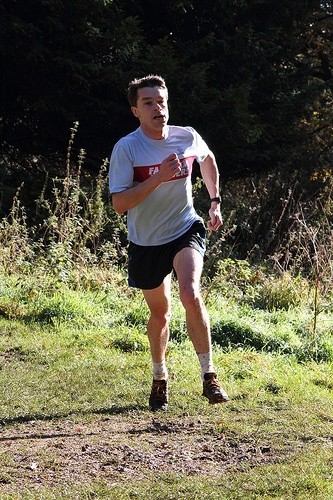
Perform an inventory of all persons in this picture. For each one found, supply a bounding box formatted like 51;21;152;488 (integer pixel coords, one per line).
109;74;229;412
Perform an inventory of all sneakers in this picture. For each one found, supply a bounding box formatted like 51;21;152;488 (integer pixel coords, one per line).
203;373;230;404
149;378;168;412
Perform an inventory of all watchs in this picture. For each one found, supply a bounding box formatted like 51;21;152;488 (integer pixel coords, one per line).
210;196;222;203
